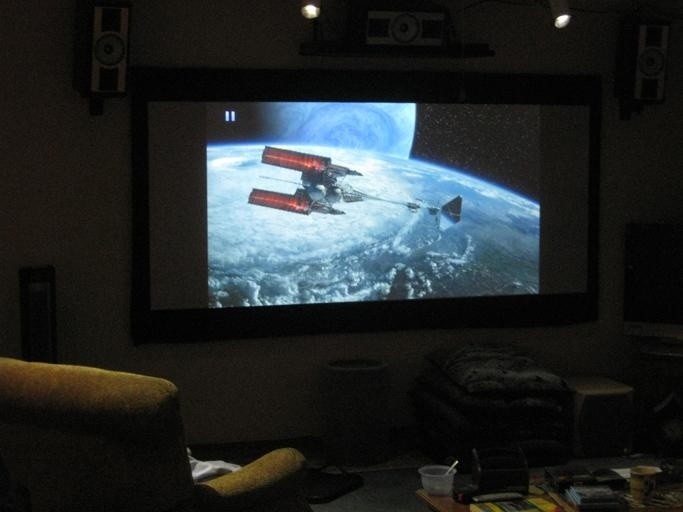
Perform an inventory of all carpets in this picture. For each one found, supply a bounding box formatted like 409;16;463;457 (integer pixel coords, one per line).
302;454;683;511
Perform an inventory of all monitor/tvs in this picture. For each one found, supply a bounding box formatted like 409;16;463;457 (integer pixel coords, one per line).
623;222;683;357
128;63;600;344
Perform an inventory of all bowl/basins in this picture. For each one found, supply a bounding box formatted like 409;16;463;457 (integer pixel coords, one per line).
418;465;457;496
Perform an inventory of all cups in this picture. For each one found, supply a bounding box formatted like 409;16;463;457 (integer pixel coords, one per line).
630;465;662;501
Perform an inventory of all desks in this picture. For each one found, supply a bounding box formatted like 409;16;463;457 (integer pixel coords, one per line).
416;476;681;511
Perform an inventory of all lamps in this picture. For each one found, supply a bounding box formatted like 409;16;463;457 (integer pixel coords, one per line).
299;1;322;19
550;1;571;29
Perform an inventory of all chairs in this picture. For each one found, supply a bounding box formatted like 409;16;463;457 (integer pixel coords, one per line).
2;359;305;511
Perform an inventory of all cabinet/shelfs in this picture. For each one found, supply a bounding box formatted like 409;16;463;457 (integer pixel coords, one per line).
626;343;682;481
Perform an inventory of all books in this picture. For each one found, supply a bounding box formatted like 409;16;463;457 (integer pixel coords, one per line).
560;485;620;511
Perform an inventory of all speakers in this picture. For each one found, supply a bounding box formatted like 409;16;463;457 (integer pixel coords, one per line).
615;18;670;105
72;1;131;99
562;372;634;458
354;5;448;52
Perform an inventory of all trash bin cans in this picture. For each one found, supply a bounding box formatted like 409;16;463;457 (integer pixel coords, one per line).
318;358;389;467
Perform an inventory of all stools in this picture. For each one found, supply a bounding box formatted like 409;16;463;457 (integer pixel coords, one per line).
563;373;633;443
